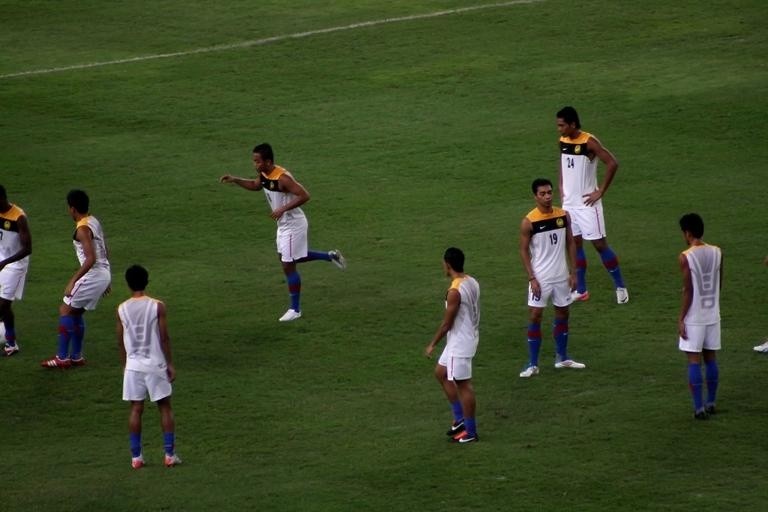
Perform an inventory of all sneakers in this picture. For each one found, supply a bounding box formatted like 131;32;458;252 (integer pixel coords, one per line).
569;289;589;301
40;355;73;368
446;417;466;436
164;453;183;467
554;359;586;369
615;285;630;305
693;403;719;420
519;366;539;378
72;352;89;366
278;308;301;322
328;249;345;272
449;430;479;444
131;453;146;469
0;342;19;357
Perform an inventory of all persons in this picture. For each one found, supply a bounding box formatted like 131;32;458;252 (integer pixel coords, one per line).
0;185;31;357
519;178;585;379
219;142;347;322
115;266;182;469
556;106;629;304
40;191;112;369
425;248;481;445
678;214;724;419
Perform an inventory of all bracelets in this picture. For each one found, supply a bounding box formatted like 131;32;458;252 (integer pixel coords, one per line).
528;278;536;281
